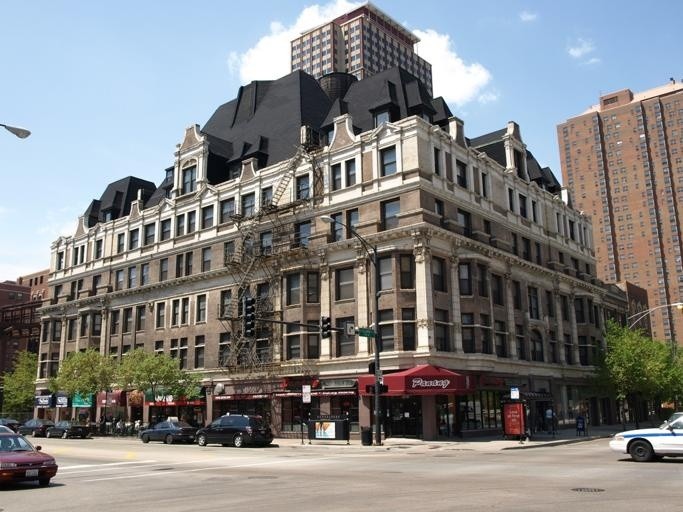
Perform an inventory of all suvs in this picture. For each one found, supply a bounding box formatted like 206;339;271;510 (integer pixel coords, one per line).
195;412;273;448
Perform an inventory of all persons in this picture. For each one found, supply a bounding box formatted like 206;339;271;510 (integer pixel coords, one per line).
115;419;122;438
546;406;559;433
134;420;140;438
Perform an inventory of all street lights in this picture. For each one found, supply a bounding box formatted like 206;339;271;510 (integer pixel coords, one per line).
320;216;384;446
626;303;683;428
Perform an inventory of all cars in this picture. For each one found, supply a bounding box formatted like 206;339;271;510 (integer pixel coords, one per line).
16;420;55;437
140;421;198;444
0;426;57;487
608;412;683;461
0;419;20;432
45;421;90;439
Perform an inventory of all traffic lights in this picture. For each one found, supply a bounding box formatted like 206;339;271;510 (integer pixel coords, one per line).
378;381;388;394
322;316;332;341
366;384;375;394
244;297;256;338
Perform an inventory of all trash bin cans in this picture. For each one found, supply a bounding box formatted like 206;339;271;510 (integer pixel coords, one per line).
361;426;373;446
